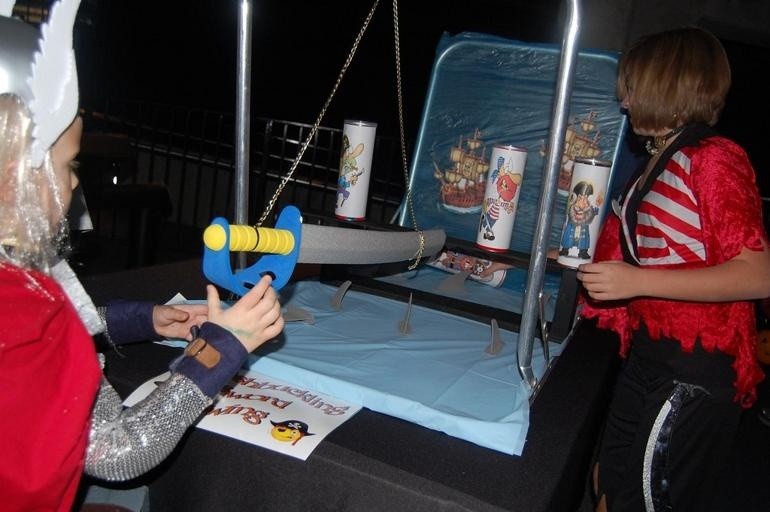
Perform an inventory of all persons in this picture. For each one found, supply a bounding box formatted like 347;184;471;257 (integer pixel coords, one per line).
497;25;768;512
0;13;287;512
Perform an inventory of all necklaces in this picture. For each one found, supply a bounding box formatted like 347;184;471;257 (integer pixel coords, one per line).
653;122;687;149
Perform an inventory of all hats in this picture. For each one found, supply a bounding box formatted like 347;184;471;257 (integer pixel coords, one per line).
0;0;81;167
